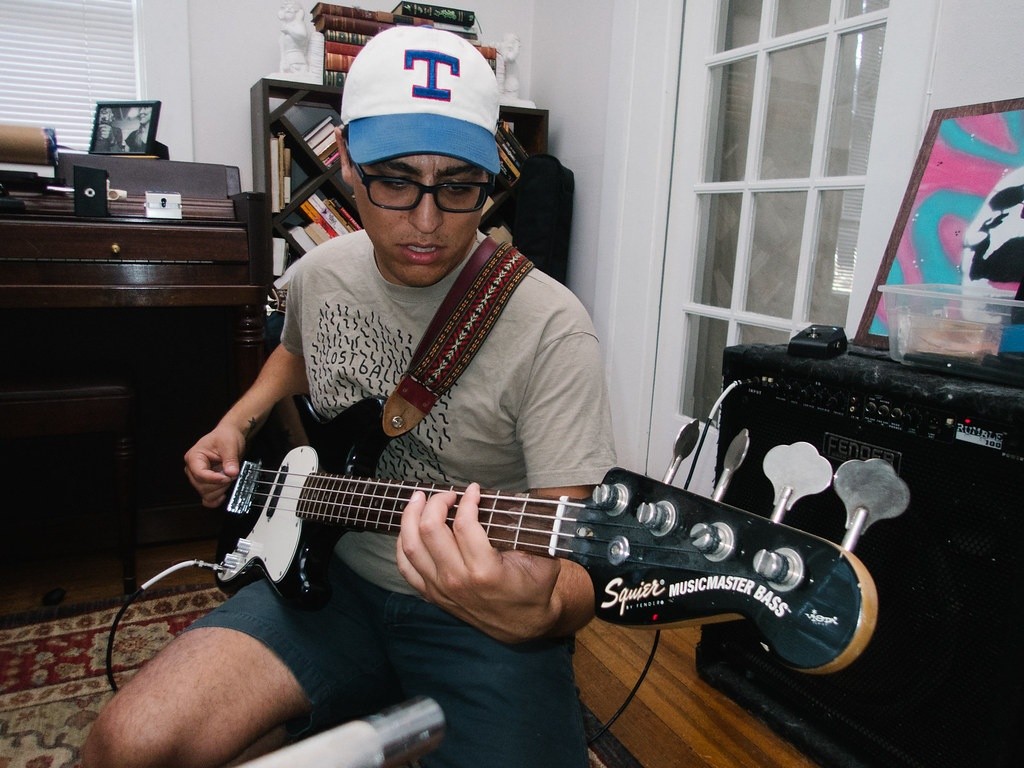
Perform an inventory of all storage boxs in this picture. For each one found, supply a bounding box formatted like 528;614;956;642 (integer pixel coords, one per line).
877;284;1024;368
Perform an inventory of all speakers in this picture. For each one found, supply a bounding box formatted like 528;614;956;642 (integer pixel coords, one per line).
696;340;1024;768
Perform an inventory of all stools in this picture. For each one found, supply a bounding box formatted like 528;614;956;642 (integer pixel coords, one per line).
0;365;148;603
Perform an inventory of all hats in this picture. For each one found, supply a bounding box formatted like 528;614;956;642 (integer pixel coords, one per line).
340;26;501;174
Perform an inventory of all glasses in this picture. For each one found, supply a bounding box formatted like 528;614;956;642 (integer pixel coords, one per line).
342;138;496;212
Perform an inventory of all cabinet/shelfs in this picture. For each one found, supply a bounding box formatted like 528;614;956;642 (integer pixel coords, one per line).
250;78;549;341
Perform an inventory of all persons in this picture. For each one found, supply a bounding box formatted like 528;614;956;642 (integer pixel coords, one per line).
81;26;619;768
95;106;152;152
489;33;521;99
277;0;308;75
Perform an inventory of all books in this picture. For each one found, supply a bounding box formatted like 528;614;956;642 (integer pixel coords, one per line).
270;0;529;290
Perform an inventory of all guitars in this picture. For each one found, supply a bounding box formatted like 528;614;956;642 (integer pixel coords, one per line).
212;393;912;676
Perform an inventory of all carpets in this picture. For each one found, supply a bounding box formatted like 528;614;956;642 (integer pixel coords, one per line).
1;579;643;768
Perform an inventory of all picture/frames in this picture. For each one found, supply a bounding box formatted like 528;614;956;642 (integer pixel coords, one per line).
854;98;1024;369
88;99;171;163
